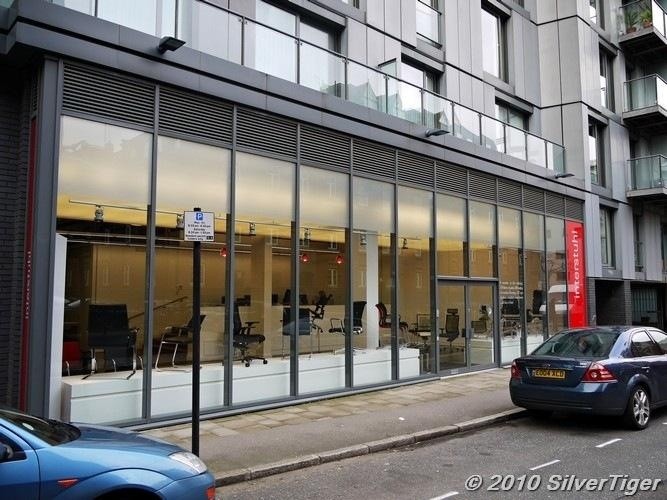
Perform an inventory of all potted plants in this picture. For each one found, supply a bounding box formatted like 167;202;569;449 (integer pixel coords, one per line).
640;10;652;29
625;10;640;34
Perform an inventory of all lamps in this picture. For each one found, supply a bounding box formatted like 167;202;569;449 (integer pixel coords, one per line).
68;199;422;248
301;255;311;263
555;173;575;179
426;129;450;137
220;247;226;257
158;36;185;54
336;256;344;264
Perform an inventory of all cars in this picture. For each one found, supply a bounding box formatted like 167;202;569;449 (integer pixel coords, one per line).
510;325;667;428
0;403;216;500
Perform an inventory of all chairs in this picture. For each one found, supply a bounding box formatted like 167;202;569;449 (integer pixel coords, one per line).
61;289;488;379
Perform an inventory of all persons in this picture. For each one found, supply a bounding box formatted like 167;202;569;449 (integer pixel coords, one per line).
317;290;328;304
283;289;290;304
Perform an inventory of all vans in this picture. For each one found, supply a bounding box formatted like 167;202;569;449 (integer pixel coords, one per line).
537;285;576;321
